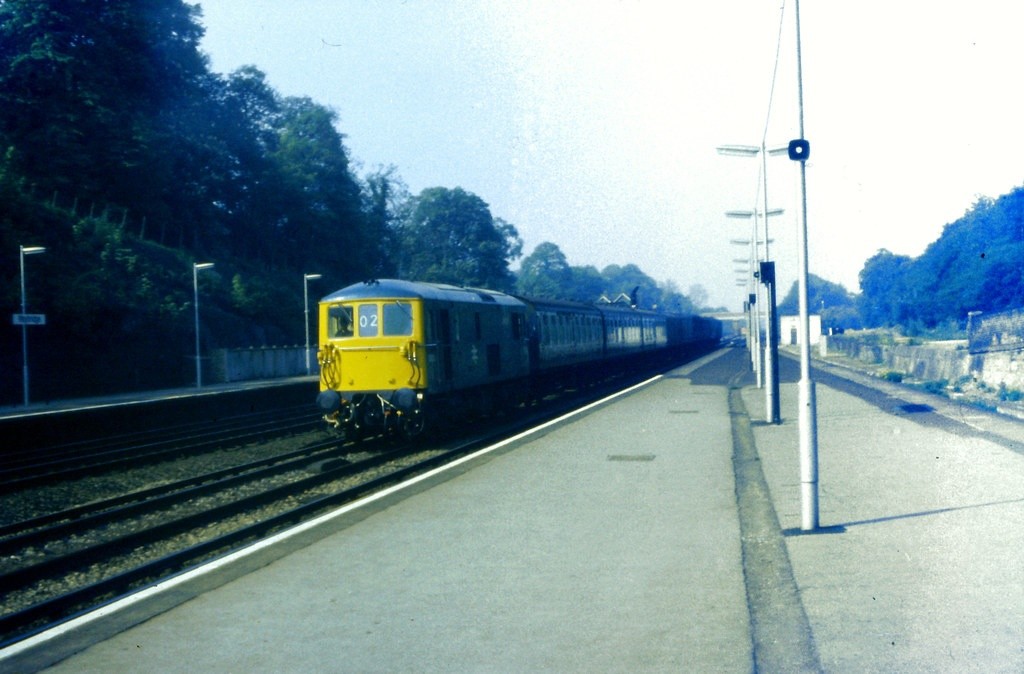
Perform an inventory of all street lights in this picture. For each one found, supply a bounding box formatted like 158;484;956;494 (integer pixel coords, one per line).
725;208;784;390
717;144;788;424
304;273;322;376
20;244;46;406
193;262;216;391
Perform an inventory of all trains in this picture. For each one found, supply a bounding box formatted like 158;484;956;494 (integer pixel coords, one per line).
317;278;724;443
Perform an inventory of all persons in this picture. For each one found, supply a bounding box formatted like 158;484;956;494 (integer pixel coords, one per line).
335;313;353;337
